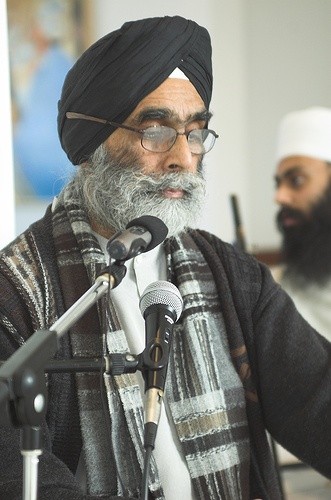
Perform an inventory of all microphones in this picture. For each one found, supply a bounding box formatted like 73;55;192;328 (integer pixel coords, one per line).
139;281;185;451
106;216;169;260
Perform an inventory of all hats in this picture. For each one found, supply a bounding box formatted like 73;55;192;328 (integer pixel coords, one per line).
57;14;214;165
276;107;331;163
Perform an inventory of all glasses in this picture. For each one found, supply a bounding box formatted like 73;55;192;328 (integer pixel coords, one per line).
66;111;219;156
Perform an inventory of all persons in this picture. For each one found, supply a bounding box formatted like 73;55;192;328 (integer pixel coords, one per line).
272;109;330;340
0;16;331;500
